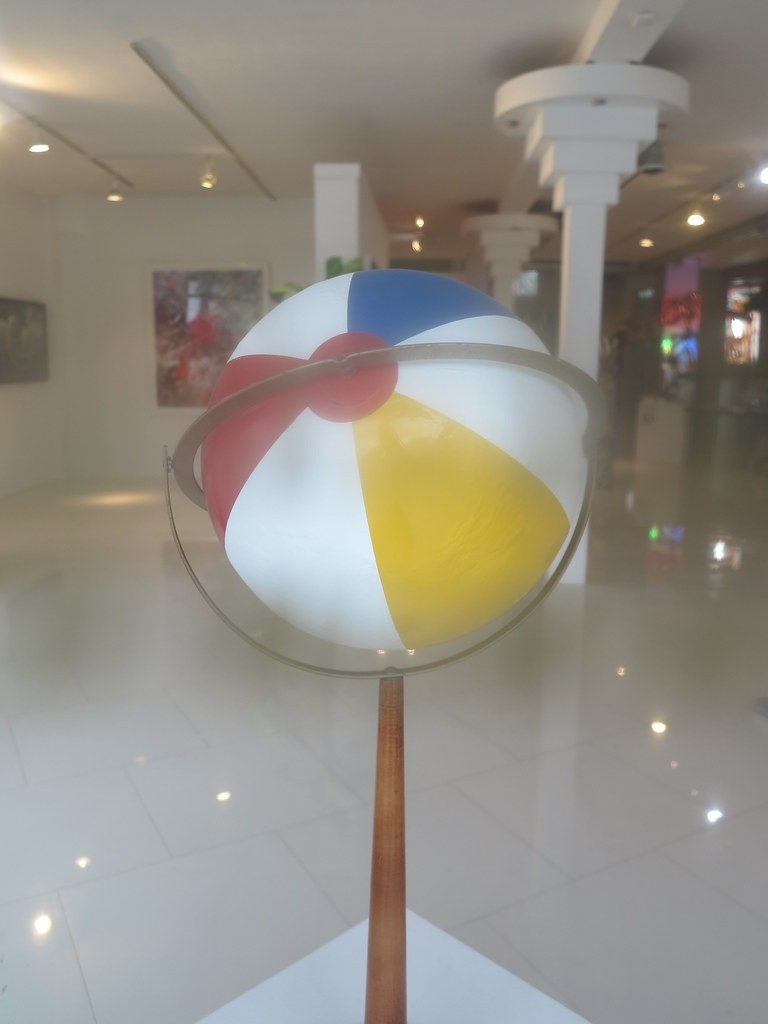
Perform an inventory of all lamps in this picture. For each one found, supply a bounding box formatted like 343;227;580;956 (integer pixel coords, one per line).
199;157;222;190
686;202;705;226
104;177;125;202
639;227;655;248
25;120;50;153
